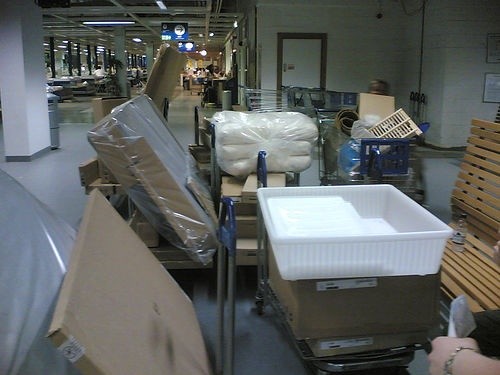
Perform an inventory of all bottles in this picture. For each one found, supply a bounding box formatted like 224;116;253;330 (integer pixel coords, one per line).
452;214;467;252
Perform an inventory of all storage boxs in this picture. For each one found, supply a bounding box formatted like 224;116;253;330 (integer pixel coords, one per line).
45;91;454;375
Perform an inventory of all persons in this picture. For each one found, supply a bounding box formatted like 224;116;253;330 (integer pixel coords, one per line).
126;64;141;71
426;240;500;375
197;68;201;75
46;64;51;76
93;66;105;88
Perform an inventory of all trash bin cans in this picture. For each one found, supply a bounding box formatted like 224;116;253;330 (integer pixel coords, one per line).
184;77;189;90
222;90;231;111
47;92;62;148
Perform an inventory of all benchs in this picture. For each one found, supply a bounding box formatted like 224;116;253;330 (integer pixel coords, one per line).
441;118;500;314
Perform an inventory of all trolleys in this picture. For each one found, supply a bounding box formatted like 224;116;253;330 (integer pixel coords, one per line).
314;106;427;207
108;105;303;375
253;150;434;375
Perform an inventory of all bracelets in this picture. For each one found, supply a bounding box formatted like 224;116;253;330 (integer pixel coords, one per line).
444;346;478;375
